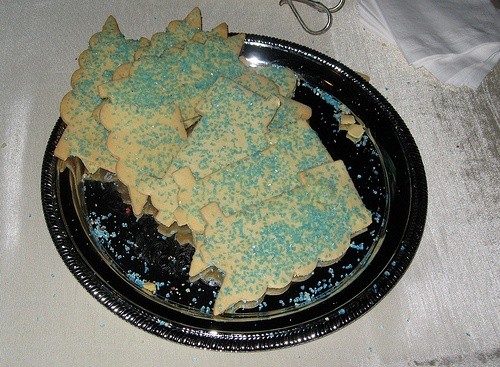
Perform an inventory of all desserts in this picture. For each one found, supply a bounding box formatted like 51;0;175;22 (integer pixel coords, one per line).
55;4;372;317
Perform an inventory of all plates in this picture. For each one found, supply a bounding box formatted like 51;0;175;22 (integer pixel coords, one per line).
40;32;429;352
